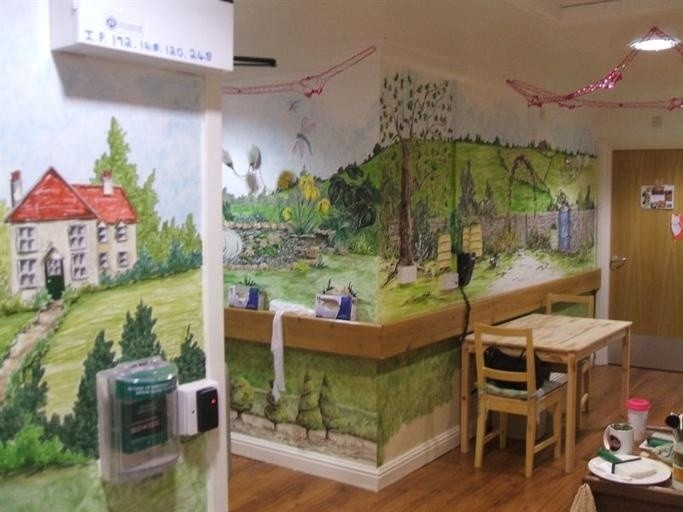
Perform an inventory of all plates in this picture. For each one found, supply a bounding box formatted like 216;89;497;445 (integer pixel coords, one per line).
587;453;672;484
639;431;673;451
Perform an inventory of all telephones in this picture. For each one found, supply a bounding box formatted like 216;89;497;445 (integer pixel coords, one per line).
457;253;475;286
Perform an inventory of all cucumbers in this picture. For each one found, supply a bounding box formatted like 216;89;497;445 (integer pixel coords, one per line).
647;437;673;448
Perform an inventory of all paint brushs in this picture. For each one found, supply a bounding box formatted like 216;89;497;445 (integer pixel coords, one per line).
666;415;681;442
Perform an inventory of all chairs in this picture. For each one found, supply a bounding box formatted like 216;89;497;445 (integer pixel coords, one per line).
474;321;566;479
541;291;596;429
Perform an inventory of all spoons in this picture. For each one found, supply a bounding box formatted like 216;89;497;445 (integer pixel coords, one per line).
665;414;681;441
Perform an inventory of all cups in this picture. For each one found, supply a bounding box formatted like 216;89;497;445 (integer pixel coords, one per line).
673;440;683;490
603;398;652;456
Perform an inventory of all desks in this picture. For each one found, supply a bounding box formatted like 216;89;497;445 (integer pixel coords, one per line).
458;312;633;476
582;423;681;512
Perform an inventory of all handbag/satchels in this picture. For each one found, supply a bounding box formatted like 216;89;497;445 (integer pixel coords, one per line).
487;348;543;390
